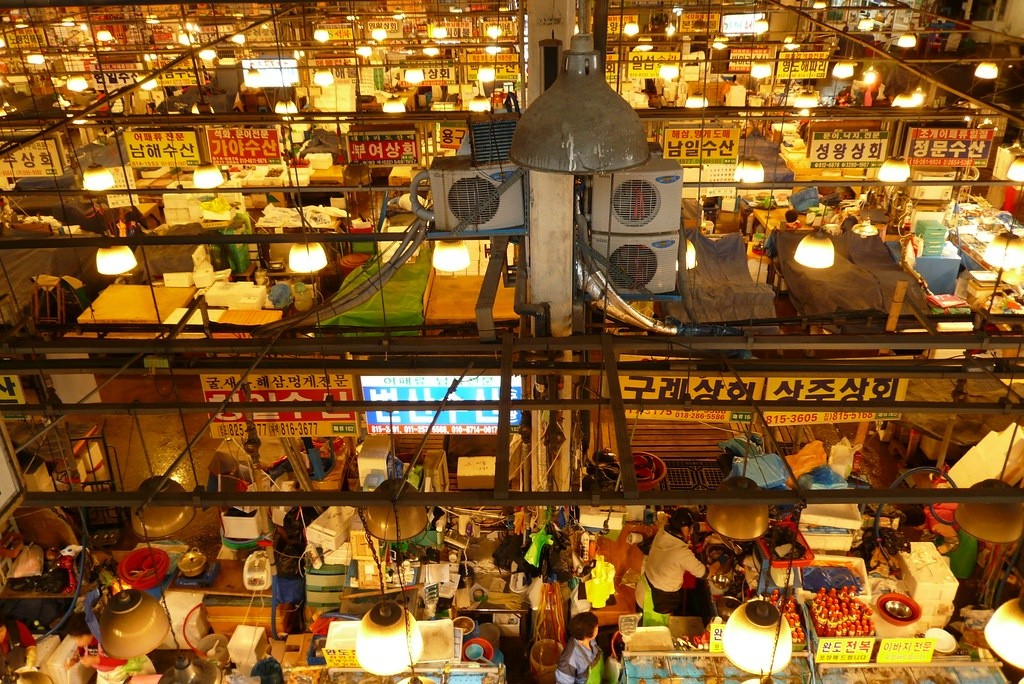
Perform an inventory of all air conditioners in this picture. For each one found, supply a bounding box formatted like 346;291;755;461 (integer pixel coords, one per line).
593;157;683;234
430;156;523;231
591;230;679;294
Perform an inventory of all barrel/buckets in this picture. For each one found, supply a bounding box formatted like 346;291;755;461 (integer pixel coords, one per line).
339;255;371;278
292;288;312;313
529;639;564;684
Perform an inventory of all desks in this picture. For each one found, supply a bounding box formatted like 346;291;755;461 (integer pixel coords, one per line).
308;164;348;197
885;241;961;295
170;556;276;598
231;263;255;281
387;166;413;198
751;207;814;238
0;549;129;599
136;202;163;227
455;570;529;666
251;216;341;277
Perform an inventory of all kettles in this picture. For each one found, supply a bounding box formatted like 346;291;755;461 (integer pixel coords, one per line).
509;573;527;592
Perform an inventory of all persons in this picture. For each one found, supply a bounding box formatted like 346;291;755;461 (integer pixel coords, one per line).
555;612;604;684
874;24;892;38
905;22;918;39
904;502;978;580
784;210;803;230
854;27;860;31
634;508;706;628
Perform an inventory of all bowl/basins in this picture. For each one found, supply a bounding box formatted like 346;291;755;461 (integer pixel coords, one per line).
454;616;476;636
197;634;230;668
885;600;912;618
876;593;924;626
349;224;375;234
631;452;667;491
462;638;493;666
925;627;957;653
118;547;170;587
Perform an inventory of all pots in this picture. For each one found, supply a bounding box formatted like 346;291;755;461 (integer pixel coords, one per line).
717;596;742;620
178;548;207;578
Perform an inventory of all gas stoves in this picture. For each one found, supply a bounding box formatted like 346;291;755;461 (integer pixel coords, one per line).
173;560;221;587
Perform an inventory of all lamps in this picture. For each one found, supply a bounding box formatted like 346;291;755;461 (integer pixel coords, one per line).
129;410;194;539
794;185;835;268
877;121;910;182
706;409;770;541
721;502;791;684
982;151;1024;285
287;240;329;272
506;0;652;176
431;240;470;270
624;0;927;108
99;509;169;659
975;33;999;79
365;478;430;539
0;15;333;191
352;505;423;676
346;6;502;113
732;118;766;185
956;413;1024;673
96;243;139;274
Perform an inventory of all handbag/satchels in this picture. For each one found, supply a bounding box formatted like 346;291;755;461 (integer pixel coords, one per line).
733;452;788;488
829;436;862;479
799;465;848;490
783;440;827;489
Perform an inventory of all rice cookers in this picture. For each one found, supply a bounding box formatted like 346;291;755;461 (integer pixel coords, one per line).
244;550;272;591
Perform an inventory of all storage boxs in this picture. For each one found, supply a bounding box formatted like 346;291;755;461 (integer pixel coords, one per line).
159;506;384;670
23;441;109;492
760;501;960;647
458;454;497;489
916;219;1024;303
492;613;520;638
134;125;333;230
219;434;496;616
162;244;265;311
